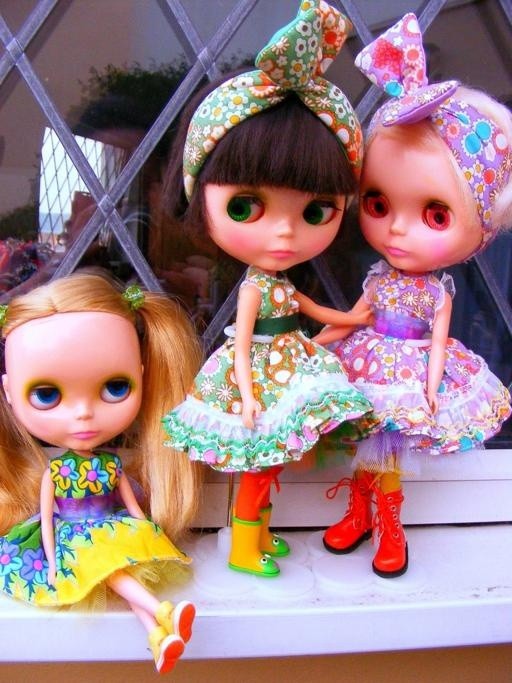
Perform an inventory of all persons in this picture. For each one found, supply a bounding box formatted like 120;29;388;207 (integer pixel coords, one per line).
162;1;378;584
0;270;204;675
308;10;512;582
